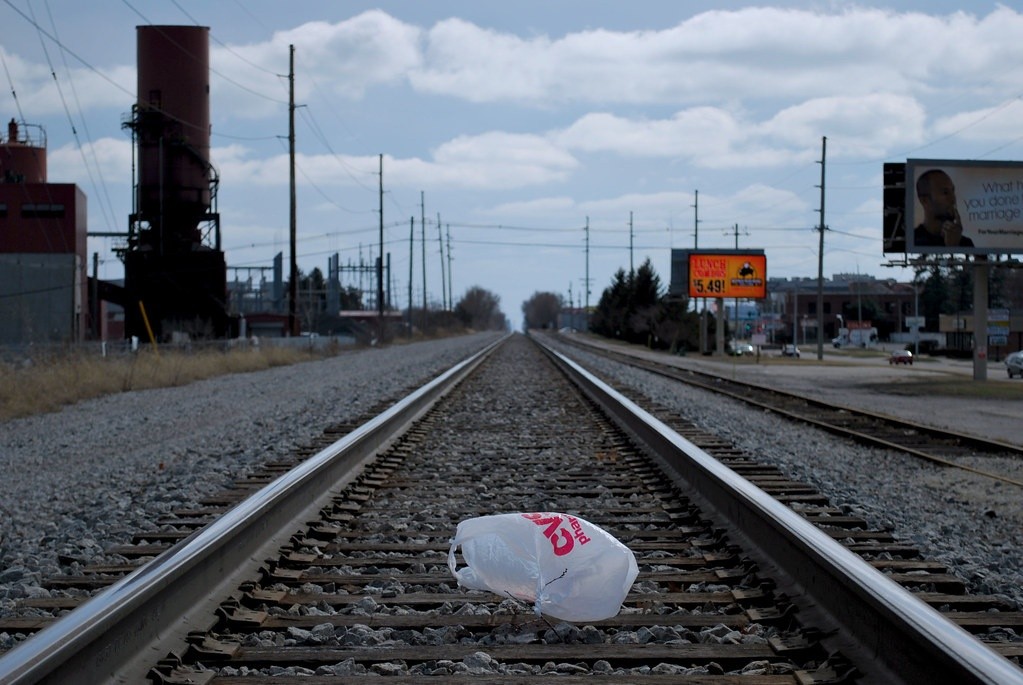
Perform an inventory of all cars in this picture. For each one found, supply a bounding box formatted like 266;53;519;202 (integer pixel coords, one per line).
1004;350;1023;379
783;345;800;358
889;350;913;365
728;337;753;357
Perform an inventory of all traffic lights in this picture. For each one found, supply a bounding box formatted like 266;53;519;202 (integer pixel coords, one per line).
757;325;762;333
745;323;751;333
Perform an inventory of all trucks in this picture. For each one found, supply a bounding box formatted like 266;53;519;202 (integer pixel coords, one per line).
832;328;878;349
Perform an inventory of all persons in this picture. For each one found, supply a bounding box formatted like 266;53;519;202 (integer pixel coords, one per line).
913;170;975;248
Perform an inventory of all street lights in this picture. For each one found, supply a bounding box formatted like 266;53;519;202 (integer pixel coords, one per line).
803;315;808;345
837;314;843;349
905;284;918;361
